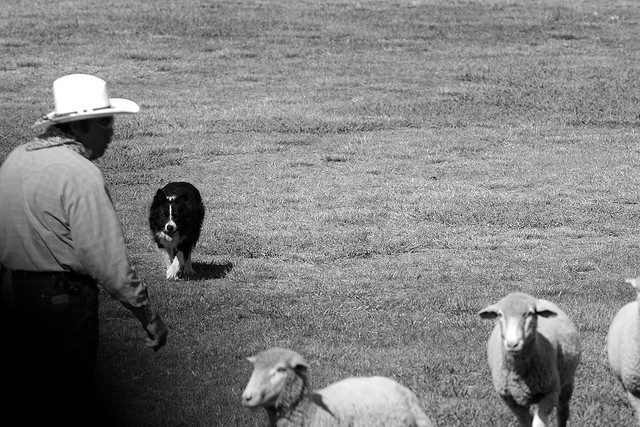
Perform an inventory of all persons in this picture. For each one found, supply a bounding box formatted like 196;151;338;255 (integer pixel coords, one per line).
0;73;168;425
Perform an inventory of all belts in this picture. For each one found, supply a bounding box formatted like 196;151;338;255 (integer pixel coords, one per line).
9;269;91;284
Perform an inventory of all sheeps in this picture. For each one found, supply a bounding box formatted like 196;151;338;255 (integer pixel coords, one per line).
478;291;582;427
606;275;640;414
241;347;434;427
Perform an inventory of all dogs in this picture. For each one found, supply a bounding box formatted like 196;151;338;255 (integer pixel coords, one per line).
149;181;205;280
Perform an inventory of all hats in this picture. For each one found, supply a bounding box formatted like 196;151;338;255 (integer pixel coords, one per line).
29;74;139;127
